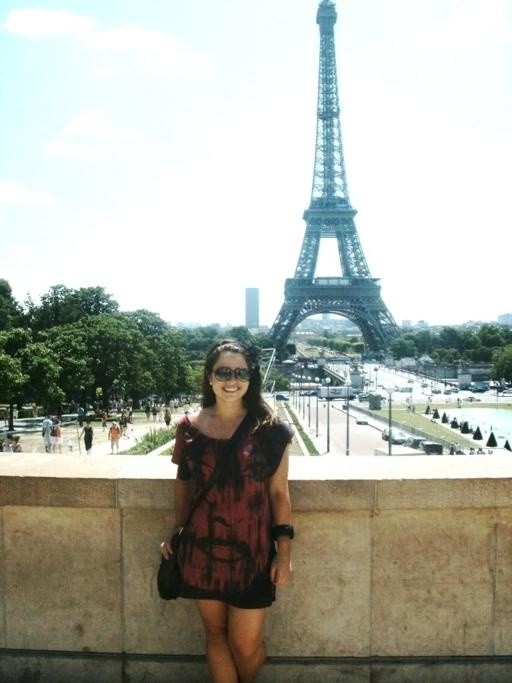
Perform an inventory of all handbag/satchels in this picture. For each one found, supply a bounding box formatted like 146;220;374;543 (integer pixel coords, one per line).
157;530;182;600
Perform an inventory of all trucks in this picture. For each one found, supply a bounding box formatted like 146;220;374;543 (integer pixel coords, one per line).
317;385;356;401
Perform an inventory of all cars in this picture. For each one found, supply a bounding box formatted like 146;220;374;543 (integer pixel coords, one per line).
344;359;378;373
298;389;316;397
353;389;387;402
395;377;512;403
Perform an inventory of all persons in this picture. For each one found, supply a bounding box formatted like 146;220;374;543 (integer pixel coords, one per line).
160;339;296;683
0;394;192;453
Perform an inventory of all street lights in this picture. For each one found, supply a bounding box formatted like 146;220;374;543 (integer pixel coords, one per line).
325;377;331;453
291;372;312;430
343;379;353;456
383;382;395;456
313;377;319;436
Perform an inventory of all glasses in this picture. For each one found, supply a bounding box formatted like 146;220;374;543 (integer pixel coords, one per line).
211;367;251;383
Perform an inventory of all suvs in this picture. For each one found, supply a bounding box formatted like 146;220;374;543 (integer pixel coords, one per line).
274;394;290;401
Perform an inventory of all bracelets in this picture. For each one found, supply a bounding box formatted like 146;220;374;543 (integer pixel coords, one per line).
271;523;295;540
